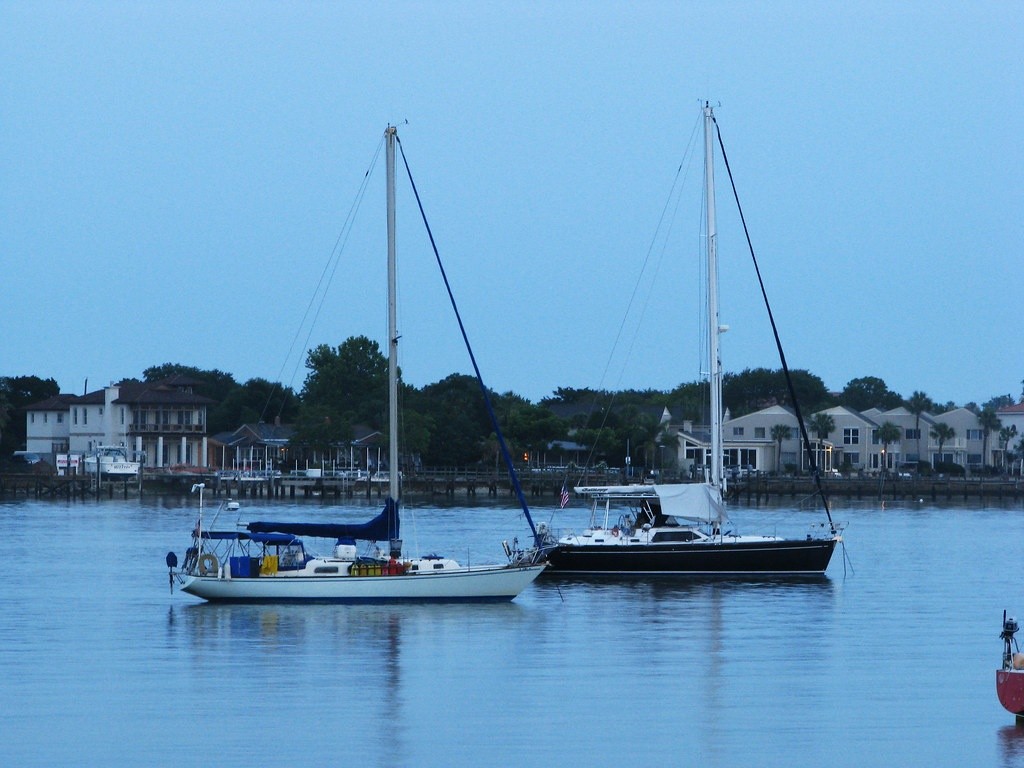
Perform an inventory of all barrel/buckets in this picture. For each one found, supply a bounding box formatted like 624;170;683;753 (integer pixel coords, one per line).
249;557;259;578
230;556;252;578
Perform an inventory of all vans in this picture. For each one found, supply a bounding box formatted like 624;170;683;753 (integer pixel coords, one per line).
12;451;42;468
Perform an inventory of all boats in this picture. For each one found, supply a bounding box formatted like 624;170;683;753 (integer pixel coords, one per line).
995;609;1023;714
83;440;140;475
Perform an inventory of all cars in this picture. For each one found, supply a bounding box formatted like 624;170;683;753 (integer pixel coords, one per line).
213;468;398;483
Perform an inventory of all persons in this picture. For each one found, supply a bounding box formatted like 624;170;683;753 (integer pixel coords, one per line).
713;522;720;534
655;467;659;484
732;466;737;478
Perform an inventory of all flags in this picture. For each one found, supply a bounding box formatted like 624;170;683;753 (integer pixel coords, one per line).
195;518;200;536
561;479;569;509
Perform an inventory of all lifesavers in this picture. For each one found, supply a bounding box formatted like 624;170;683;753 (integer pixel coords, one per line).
199;554;218;573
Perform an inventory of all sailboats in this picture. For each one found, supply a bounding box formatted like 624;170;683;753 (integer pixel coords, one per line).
502;93;848;576
164;119;552;603
